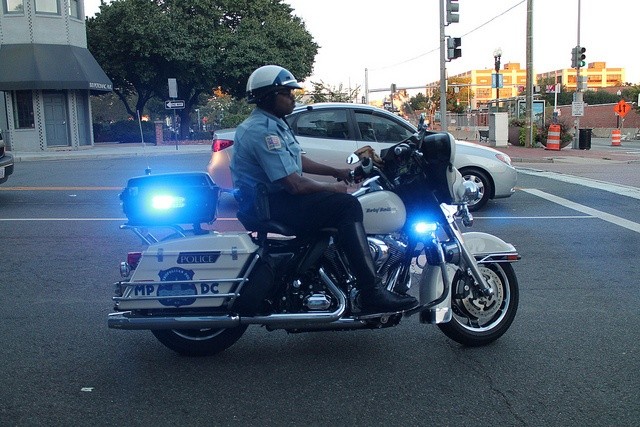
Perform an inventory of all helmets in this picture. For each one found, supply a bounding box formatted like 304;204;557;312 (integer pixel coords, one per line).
246;64;304;104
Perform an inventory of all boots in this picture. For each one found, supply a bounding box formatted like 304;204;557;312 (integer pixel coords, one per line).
339;220;418;313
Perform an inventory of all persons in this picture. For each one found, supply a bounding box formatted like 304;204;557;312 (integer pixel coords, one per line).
230;65;418;313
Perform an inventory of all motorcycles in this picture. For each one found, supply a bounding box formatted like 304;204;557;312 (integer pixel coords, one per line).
107;114;521;356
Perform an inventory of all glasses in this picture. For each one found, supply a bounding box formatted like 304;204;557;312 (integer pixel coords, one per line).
274;90;290;98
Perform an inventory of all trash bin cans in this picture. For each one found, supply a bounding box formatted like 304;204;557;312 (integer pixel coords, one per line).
579;128;592;150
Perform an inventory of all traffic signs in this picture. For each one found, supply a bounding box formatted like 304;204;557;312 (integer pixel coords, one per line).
165;100;185;109
572;102;583;116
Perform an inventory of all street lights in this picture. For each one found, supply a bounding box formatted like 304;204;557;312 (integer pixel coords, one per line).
195;109;200;131
617;90;621;128
493;48;501;106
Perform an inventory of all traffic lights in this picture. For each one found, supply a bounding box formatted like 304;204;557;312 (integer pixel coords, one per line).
447;38;461;58
446;0;459;22
577;46;586;67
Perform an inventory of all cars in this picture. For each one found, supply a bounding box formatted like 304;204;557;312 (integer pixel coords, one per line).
208;103;516;210
